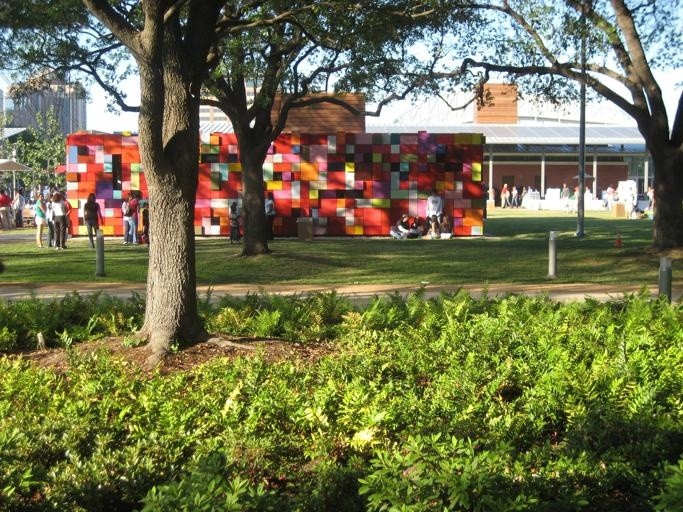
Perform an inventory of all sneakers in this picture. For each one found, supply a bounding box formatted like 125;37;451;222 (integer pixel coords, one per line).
39;238;149;252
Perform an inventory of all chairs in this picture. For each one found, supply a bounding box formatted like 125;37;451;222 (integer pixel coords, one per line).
523;187;637;216
21;207;37;227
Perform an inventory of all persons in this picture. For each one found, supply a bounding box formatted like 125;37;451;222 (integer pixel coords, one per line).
389;187;452;240
0;185;277;249
486;181;654;212
